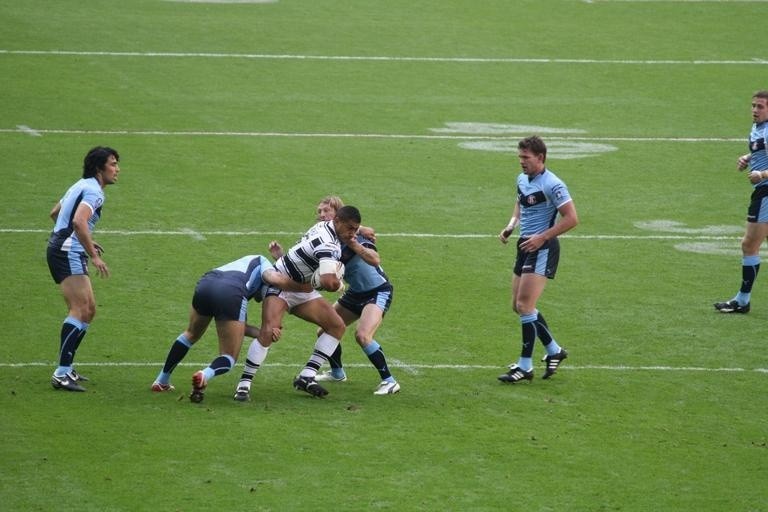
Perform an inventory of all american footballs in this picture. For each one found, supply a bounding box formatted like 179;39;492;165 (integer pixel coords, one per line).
311;261;345;292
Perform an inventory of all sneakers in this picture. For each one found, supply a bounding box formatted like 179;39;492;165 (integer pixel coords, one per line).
70;369;89;381
151;381;175;392
542;347;568;379
314;371;347;382
233;386;250;401
497;363;534;384
51;372;85;392
293;375;329;396
714;299;750;313
190;370;209;401
373;380;400;396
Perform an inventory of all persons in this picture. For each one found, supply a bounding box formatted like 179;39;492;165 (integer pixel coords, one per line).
233;206;361;401
496;136;578;382
152;255;314;404
713;91;768;314
268;196;402;395
46;146;119;393
